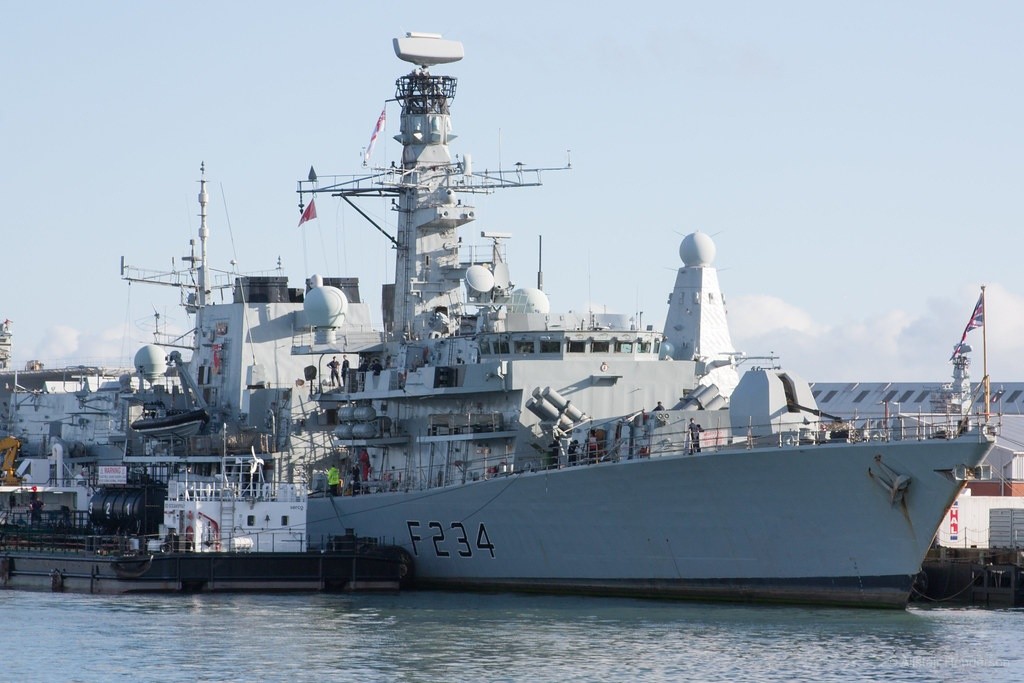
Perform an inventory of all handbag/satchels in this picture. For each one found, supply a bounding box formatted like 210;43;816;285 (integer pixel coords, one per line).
366;461;370;468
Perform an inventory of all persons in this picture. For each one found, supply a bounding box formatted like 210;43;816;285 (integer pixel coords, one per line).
372;358;383;376
351;462;360;497
585;430;598;464
341;354;349;386
327;356;342;388
688;417;701;455
652;401;664;411
327;464;339;496
360;449;370;481
548;435;560;467
568;440;579;467
29;496;46;526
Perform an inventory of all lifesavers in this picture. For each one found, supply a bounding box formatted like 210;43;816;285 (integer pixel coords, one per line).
186;526;193;540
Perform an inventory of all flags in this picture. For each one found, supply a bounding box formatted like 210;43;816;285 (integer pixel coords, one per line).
366;109;385;155
949;293;985;362
298;199;317;227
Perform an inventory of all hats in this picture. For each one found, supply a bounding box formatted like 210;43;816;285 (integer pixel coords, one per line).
362;448;367;451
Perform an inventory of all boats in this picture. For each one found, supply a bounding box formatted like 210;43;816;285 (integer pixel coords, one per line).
0;30;999;610
